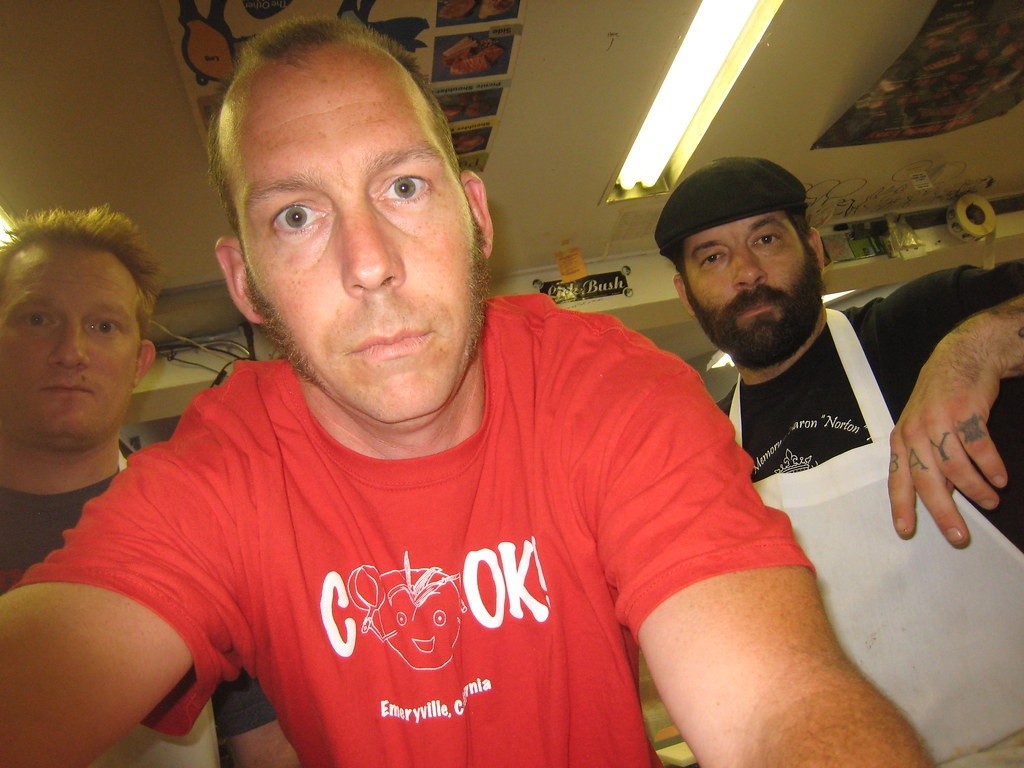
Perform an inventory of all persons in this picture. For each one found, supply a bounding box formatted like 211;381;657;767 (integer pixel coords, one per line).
0;202;303;768
0;16;936;768
656;156;1024;768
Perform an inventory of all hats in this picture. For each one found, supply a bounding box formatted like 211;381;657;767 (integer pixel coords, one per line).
654;155;806;259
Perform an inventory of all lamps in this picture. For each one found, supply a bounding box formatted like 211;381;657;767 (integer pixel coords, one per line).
597;0;785;208
